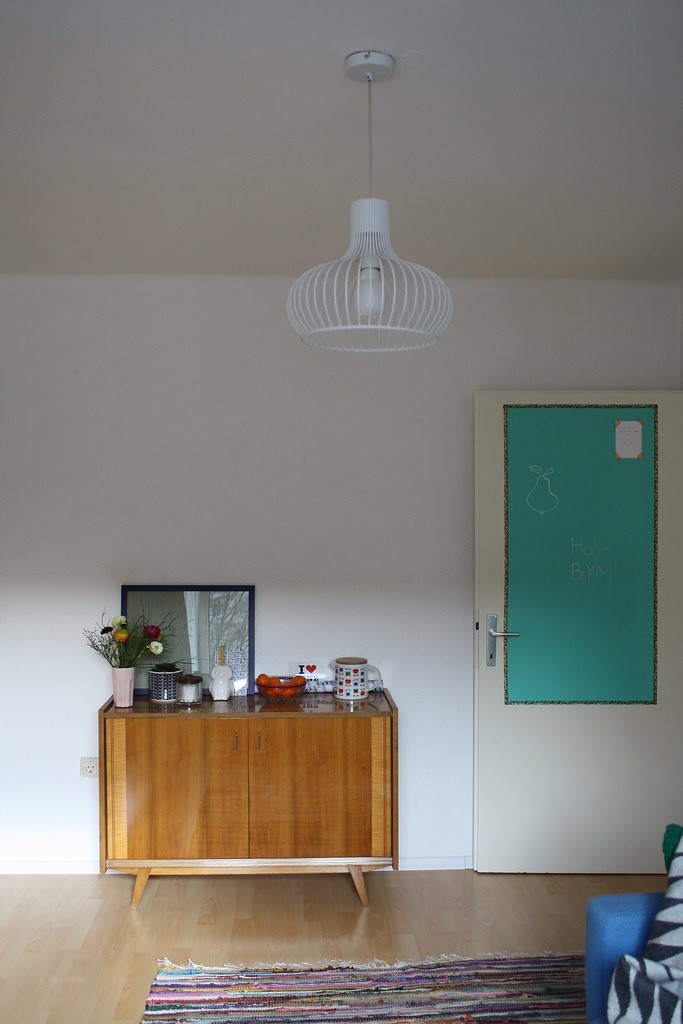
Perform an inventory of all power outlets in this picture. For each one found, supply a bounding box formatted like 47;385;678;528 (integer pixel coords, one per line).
79;757;98;778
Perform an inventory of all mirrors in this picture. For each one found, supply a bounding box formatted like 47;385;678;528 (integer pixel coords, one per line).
120;584;257;698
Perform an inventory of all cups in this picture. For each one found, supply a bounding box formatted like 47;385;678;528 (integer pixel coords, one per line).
333;657;381;700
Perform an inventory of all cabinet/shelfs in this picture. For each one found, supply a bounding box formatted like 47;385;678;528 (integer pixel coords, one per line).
97;687;400;911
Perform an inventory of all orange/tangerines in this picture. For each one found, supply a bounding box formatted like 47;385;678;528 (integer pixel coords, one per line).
257;674;306;698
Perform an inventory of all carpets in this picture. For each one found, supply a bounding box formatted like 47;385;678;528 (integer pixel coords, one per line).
139;949;585;1024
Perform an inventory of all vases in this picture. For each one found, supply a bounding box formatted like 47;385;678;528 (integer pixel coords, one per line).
111;667;136;710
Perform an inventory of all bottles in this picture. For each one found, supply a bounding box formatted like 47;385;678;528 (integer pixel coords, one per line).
175;674;203;705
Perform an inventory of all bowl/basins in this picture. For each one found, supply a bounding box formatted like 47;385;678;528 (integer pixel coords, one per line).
256;676;306;703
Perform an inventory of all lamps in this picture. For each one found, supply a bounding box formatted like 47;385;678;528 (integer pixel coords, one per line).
283;52;457;354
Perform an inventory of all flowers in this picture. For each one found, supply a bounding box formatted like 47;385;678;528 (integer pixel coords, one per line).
81;610;197;669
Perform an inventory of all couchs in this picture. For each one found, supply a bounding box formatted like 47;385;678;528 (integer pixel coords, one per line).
583;824;683;1024
